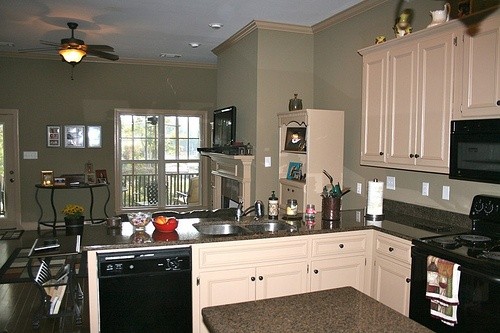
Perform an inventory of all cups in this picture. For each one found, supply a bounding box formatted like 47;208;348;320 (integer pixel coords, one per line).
107;217;122;228
321;197;341;221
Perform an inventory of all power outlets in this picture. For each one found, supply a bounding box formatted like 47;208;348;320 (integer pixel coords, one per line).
23;151;38;160
386;177;395;190
265;156;272;168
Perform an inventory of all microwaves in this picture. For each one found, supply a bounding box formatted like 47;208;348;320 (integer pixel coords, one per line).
449;117;500;185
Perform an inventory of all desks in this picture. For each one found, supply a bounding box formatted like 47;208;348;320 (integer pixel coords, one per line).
35;183;111;237
202;286;435;333
27;235;84;330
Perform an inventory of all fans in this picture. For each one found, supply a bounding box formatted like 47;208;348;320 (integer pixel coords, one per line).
39;22;120;61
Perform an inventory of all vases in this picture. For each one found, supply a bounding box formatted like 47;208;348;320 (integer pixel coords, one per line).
64;216;84;227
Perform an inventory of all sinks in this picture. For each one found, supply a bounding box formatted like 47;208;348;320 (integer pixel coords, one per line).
243;222;297;232
192;222;246;234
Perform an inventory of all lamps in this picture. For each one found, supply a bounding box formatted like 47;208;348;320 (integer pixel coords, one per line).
59;42;88;64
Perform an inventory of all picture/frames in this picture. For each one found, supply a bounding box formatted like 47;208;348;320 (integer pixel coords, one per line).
47;124;103;148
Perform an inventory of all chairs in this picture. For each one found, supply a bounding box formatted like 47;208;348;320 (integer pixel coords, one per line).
135;182;158;206
176;177;199;205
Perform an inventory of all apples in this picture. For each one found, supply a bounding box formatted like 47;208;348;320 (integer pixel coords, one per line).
155;216;176;224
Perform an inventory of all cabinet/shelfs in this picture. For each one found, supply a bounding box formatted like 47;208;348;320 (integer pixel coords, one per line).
358;5;500;173
192;229;414;333
277;109;345;213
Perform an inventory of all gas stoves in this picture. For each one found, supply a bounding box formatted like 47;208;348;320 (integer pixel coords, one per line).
411;194;500;265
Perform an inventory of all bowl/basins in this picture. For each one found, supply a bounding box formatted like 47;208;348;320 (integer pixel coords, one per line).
151;216;178;232
127;212;152;231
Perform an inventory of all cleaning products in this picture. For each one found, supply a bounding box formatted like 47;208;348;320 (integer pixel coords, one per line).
268;190;279;220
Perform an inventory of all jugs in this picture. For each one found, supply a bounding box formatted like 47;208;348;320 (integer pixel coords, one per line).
426;3;451;28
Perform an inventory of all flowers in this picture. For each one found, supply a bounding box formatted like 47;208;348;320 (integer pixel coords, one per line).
62;204;85;218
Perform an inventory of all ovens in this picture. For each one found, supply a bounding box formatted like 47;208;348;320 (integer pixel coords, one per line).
408;238;500;333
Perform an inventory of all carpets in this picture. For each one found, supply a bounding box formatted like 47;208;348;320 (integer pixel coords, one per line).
0;247;77;284
0;230;24;241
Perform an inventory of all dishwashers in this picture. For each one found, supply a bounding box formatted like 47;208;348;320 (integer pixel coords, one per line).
95;244;193;333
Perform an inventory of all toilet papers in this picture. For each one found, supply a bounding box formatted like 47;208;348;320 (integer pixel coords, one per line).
367;180;384;216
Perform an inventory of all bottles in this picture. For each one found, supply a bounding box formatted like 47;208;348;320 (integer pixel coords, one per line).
238;143;253;155
289;94;303;111
305;205;316;216
287;199;298;216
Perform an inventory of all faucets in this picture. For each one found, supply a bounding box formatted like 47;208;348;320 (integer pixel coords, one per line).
234;197;265;222
